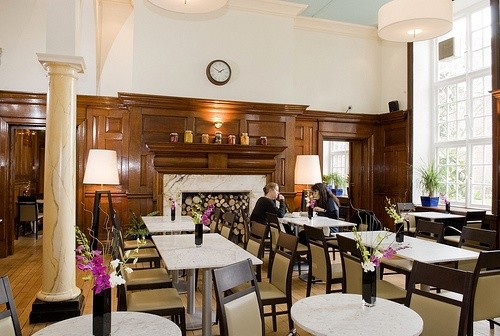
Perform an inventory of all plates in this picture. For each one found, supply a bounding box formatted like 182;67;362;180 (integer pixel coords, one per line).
291;216;301;218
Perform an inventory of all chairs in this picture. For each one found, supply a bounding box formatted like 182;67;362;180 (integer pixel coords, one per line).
71;211;186;334
209;199;499;336
14;195;43;240
0;276;23;336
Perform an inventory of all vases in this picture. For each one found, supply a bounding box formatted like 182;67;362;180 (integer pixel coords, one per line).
362;266;377;307
308;205;314;218
445;202;451;214
170;206;176;221
92;284;112;336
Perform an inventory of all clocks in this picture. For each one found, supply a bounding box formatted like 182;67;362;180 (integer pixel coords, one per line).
205;60;231;86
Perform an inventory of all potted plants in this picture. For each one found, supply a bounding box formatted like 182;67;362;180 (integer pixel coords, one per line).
321;172;344;195
384;189;409;243
402;161;442;207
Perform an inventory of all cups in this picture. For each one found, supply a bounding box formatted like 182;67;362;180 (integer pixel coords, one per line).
313;212;318;217
293;212;299;216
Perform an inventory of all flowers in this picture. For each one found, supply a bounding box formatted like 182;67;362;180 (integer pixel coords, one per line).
163;190;182;209
440;193;449;204
191;203;215;225
353;207;410;273
304;183;316;208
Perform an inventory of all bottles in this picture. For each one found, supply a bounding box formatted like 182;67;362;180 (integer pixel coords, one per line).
241;133;249;145
228;134;235;144
202;133;209;143
169;133;179;142
184;131;194;143
260;136;267;145
215;131;222;144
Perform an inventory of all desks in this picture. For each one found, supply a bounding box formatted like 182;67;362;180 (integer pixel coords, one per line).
32;212;500;336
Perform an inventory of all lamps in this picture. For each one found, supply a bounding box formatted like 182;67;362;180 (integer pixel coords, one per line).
293;155;322;194
80;149;120;191
377;0;453;42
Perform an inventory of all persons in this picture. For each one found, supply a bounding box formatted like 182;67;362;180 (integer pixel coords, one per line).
250;183;306;264
299;183;339;257
